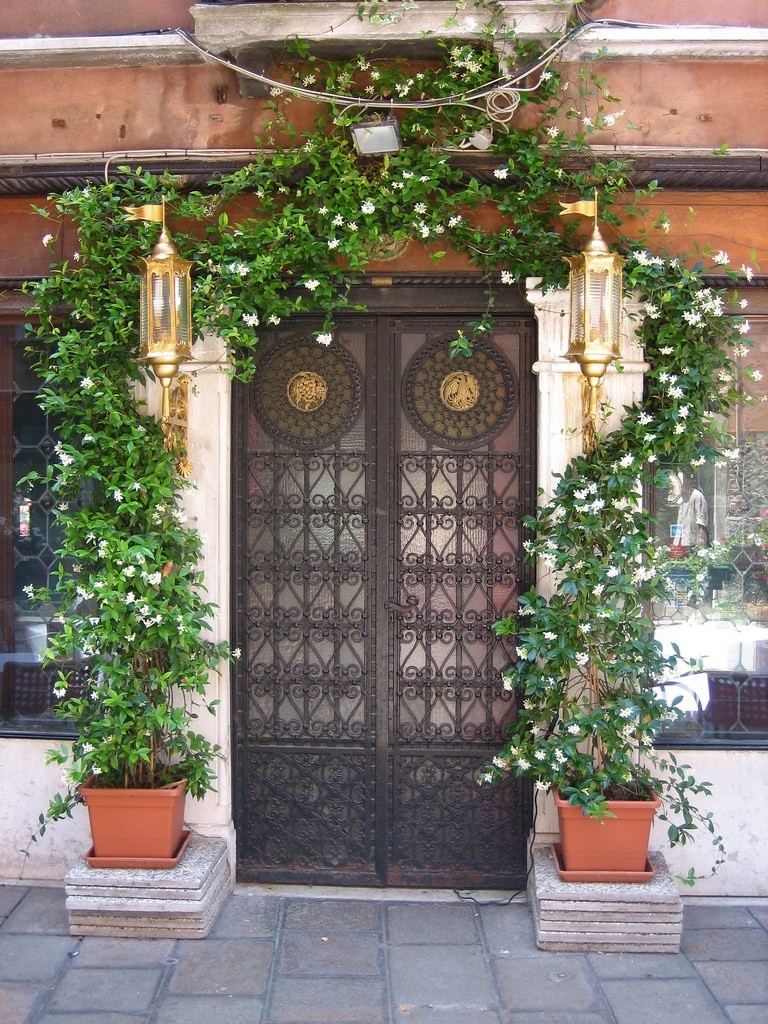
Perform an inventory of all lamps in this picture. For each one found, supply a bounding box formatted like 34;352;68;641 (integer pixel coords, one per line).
559;188;630;464
350;117;404;157
123;195;197;478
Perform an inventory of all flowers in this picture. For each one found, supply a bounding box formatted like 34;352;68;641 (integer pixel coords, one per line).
22;0;768;882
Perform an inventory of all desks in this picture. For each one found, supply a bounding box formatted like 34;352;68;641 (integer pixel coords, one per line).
0;653;94;673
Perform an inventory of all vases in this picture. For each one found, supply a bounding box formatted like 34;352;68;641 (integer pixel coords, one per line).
80;773;193;868
709;566;729;589
551;780;661;883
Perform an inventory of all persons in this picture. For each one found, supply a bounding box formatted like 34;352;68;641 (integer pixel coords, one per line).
674;477;709;553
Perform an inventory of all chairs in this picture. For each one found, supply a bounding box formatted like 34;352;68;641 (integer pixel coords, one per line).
707;669;768;733
0;654;101;723
